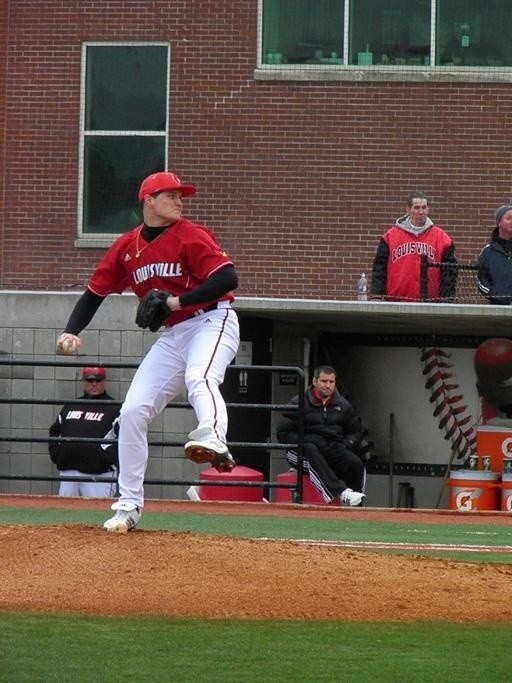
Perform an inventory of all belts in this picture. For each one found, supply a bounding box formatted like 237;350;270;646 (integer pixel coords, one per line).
189;303;217;319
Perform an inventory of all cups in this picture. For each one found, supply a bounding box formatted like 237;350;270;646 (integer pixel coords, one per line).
468;454;479;470
481;455;490;472
503;458;512;475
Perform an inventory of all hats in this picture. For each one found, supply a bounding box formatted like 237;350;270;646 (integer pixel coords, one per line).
82;367;106;381
138;172;196;202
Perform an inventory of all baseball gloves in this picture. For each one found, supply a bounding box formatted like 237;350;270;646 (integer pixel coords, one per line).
136;288;173;333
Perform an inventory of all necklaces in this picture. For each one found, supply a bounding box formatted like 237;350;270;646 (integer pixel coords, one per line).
136;220;170;258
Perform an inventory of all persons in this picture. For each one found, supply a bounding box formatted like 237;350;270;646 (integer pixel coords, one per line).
373;191;455;303
48;366;122;501
276;362;368;509
58;171;240;535
473;201;512;308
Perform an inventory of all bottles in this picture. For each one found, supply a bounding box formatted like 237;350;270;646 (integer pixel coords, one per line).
356;270;367;300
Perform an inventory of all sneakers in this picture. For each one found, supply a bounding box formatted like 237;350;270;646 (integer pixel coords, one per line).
104;501;141;531
184;437;236;472
339;488;367;506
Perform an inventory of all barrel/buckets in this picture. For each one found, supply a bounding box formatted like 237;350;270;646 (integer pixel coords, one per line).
474;424;512;471
449;470;500;511
501;473;512;512
199;463;264;501
277;472;342;507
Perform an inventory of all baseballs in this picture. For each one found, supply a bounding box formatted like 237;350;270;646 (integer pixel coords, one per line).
59;336;76;355
